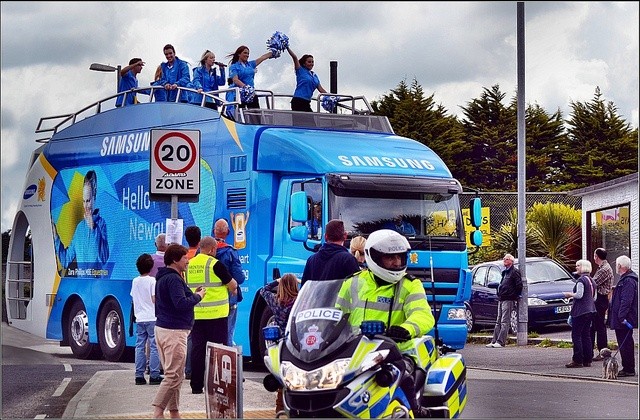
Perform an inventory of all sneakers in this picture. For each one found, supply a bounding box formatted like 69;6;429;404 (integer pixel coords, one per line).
135;377;146;384
566;362;583;368
592;354;602;361
492;342;502;347
150;376;163;385
618;371;634;377
486;343;493;347
192;388;202;394
584;363;591;366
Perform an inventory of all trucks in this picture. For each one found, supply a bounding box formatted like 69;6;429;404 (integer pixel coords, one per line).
6;86;482;361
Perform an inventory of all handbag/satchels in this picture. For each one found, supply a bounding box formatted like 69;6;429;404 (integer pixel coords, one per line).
567;315;572;327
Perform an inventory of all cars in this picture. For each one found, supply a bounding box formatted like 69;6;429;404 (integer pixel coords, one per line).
467;257;577;335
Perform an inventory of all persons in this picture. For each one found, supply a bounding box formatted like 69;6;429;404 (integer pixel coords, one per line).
335;229;437;344
132;62;165;101
563;259;597;367
182;236;237;395
196;218;245;347
260;273;302;335
192;50;226;111
486;254;523;347
226;45;275;125
115;58;144;107
383;205;416;237
608;255;638;377
284;43;331;129
152;245;206;420
52;170;109;279
160;44;192;103
349;236;368;270
304;203;322;240
129;253;164;385
592;248;614;361
150;233;169;278
184;225;202;259
300;219;361;289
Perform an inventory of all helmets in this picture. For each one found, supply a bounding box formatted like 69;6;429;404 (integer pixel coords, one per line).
364;228;412;285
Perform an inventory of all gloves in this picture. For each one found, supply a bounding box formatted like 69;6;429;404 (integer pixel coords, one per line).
386;328;408;343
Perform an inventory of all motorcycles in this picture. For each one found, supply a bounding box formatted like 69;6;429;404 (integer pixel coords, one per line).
261;278;467;419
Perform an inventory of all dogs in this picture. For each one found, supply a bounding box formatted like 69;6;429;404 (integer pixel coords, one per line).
599;347;620;380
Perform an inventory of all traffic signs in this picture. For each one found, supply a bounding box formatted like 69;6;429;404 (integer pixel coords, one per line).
150;128;200;195
